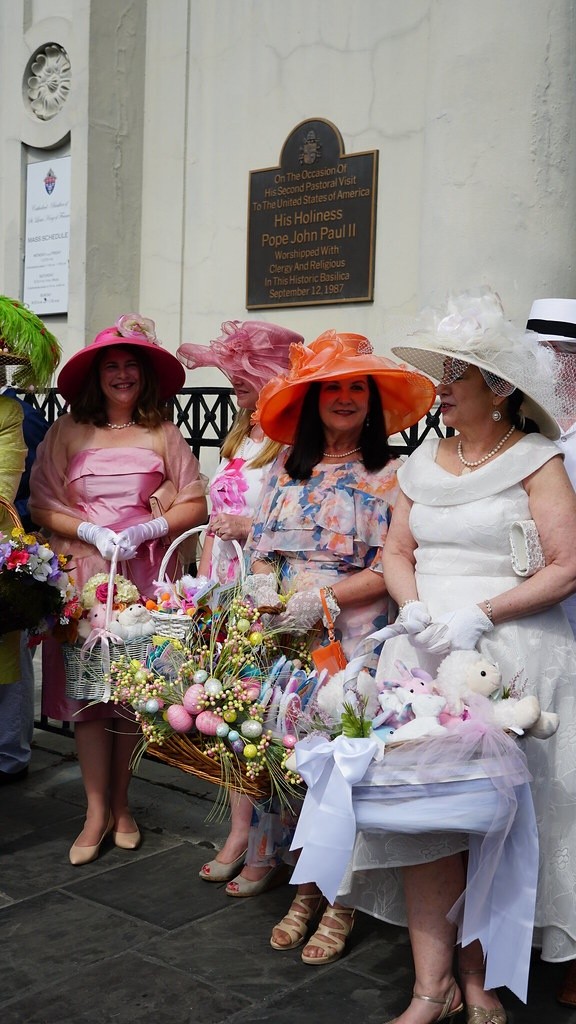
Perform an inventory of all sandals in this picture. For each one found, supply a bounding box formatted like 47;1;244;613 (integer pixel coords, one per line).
459;967;506;1024
270;891;325;949
383;982;463;1024
300;905;361;964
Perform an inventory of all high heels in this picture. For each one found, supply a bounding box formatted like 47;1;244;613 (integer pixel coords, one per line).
224;860;277;894
198;848;250;881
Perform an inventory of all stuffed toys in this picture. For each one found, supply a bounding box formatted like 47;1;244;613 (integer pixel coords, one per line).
316;649;560;740
77;604;156;645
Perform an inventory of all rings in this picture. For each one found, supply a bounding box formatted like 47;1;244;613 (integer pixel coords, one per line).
216;530;219;534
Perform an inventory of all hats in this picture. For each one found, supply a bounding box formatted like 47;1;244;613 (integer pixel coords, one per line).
0;295;64;394
178;321;304;396
249;330;435;445
357;285;576;441
57;313;186;404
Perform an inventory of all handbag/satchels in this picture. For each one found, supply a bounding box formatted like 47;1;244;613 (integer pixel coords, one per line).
311;588;348;677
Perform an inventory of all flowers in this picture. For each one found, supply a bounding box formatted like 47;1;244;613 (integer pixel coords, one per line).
152;573;209;595
0;526;83;649
81;573;141;612
288;688;373;739
502;668;529;701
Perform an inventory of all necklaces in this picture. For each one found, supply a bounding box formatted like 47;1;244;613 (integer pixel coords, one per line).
106;421;135;429
457;425;515;475
240;432;266;461
324;447;360;457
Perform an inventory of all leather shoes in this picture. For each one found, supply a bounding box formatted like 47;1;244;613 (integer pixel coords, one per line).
69;807;114;864
110;816;141;849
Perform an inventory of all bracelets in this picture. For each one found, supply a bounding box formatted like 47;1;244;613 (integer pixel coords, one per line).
485;600;492;622
399;600;416;622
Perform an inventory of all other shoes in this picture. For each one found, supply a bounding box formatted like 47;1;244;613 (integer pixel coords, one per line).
557;971;576;1005
0;764;29;785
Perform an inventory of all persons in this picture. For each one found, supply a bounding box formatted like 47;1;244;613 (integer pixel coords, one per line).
527;298;576;491
240;328;436;965
0;296;62;787
176;318;304;898
335;291;576;1024
26;311;210;865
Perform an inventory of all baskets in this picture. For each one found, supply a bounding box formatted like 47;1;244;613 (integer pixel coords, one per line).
337;623;509;839
150;524;247;640
138;602;324;800
65;541;152;699
0;495;59;634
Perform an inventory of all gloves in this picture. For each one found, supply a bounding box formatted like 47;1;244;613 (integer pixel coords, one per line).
76;522;138;560
403;600;493;655
114;517;169;551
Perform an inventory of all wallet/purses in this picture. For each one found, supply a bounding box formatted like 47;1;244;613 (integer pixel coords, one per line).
507;520;546;576
150;480;178;518
